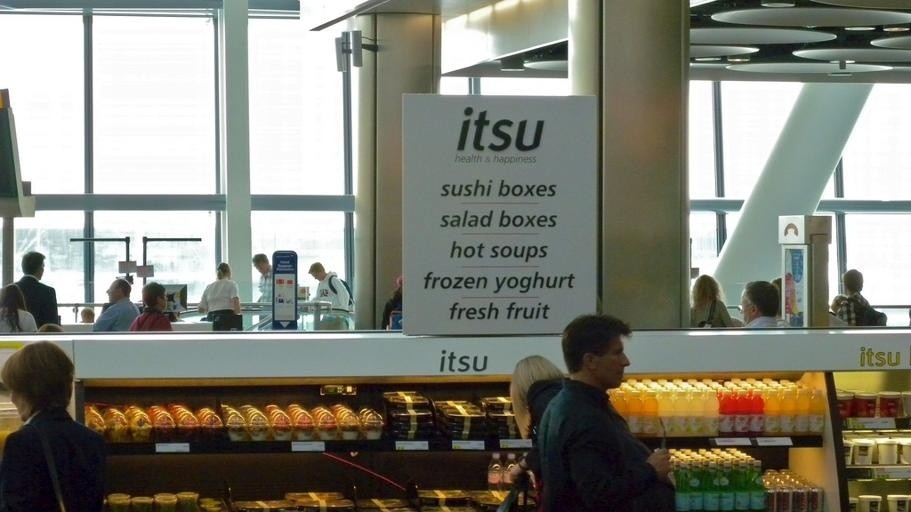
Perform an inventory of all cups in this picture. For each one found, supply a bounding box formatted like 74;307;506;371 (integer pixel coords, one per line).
849;493;911;511
836;386;911;419
104;490;223;511
843;436;911;465
298;286;311;300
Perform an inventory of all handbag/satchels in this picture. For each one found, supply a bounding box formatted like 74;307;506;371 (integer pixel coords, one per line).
697;317;721;328
495;473;531;512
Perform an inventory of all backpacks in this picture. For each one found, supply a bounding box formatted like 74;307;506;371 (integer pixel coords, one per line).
600;402;678;512
846;295;888;327
327;274;353;307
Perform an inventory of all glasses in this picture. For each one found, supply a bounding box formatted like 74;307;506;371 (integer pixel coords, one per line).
736;303;754;314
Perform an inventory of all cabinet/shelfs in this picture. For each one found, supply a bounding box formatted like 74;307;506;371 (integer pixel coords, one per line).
0;331;911;512
176;300;352;333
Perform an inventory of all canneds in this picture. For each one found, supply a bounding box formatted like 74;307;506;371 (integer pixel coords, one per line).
762;468;822;512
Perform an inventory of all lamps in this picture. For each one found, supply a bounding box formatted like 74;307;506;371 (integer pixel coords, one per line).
500;1;911;78
354;29;381;74
334;37;355;71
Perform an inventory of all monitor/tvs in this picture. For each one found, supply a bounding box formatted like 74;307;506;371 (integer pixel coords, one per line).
158;285;187;322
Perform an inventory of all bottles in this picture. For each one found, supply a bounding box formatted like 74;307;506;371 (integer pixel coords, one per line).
611;378;827;438
488;452;536;491
667;447;766;511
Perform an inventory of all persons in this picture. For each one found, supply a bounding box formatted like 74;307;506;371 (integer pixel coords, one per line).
536;315;672;512
81;309;95;323
40;323;62;331
0;340;107;511
510;355;675;511
15;251;58;329
252;254;273;330
198;263;243;331
691;269;871;327
0;284;38;331
309;262;349;329
381;277;402;329
93;279;141;331
128;281;172;330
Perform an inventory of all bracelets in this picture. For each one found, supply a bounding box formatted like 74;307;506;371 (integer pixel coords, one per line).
518;456;530;471
